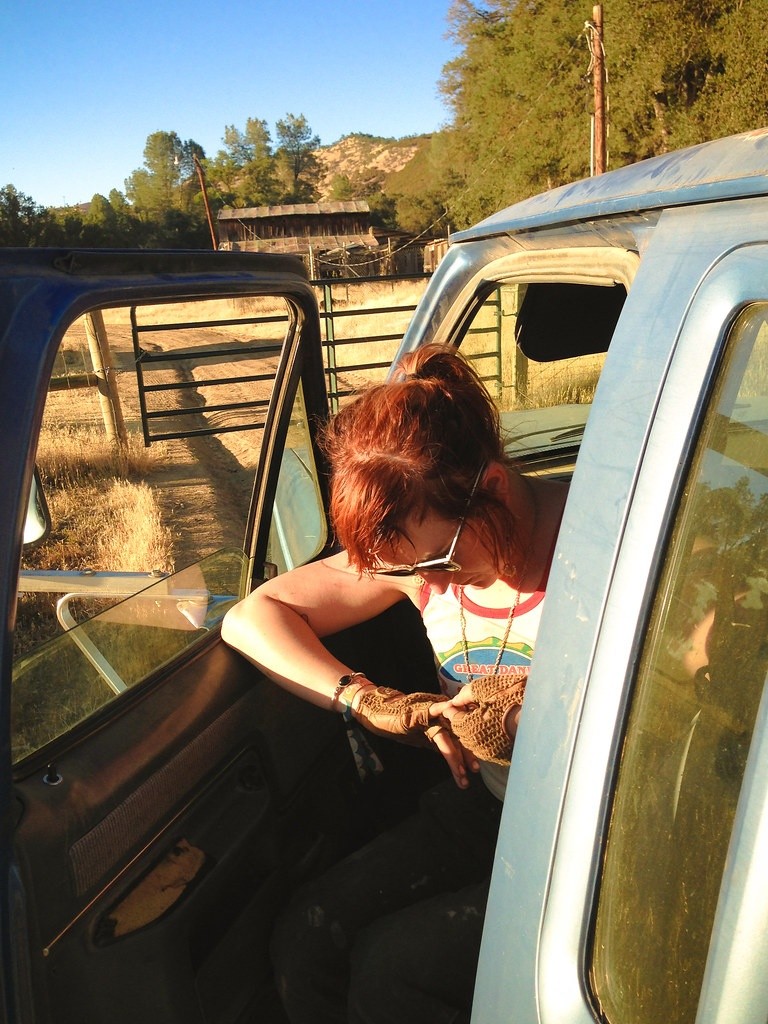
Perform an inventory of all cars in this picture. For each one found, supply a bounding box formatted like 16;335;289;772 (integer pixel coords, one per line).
0;126;768;1023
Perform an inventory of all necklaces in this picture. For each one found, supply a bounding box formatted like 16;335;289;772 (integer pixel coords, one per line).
457;475;539;682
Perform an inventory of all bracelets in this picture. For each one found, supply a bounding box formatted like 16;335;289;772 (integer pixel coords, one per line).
336;679;384;718
330;672;366;713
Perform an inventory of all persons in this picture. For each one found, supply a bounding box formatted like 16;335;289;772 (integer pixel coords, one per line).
220;345;728;1024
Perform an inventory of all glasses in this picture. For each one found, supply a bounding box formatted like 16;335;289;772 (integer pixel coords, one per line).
367;460;486;576
